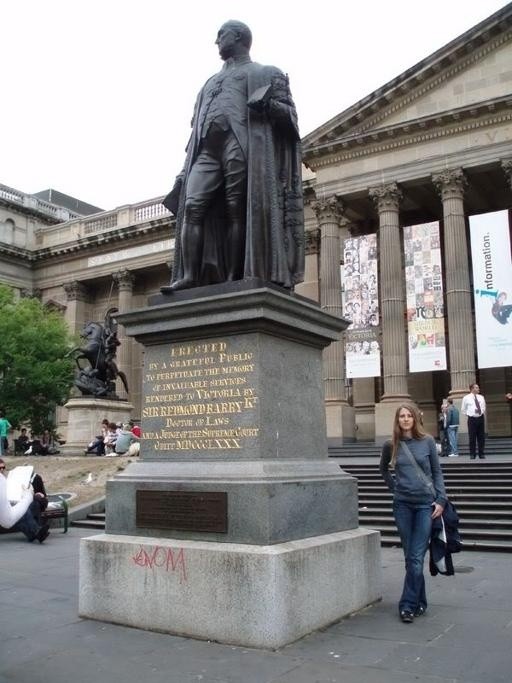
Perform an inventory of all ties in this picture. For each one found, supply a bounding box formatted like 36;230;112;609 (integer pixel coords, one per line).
474;396;481;415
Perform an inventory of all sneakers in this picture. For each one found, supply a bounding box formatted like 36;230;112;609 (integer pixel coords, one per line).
400;604;426;623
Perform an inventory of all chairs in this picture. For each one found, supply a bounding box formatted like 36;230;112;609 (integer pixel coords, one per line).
41;496;69;535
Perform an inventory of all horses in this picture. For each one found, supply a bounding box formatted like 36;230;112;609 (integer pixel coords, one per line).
62;320;130;395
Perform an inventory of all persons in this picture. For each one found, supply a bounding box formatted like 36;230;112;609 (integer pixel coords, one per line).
39;428;55;456
19;464;49;543
0;411;13;456
1;459;49;544
340;221;446;354
158;15;299;296
435;402;450;456
81;417;140;457
17;427;33;456
443;397;459;457
378;402;448;624
459;382;487;458
